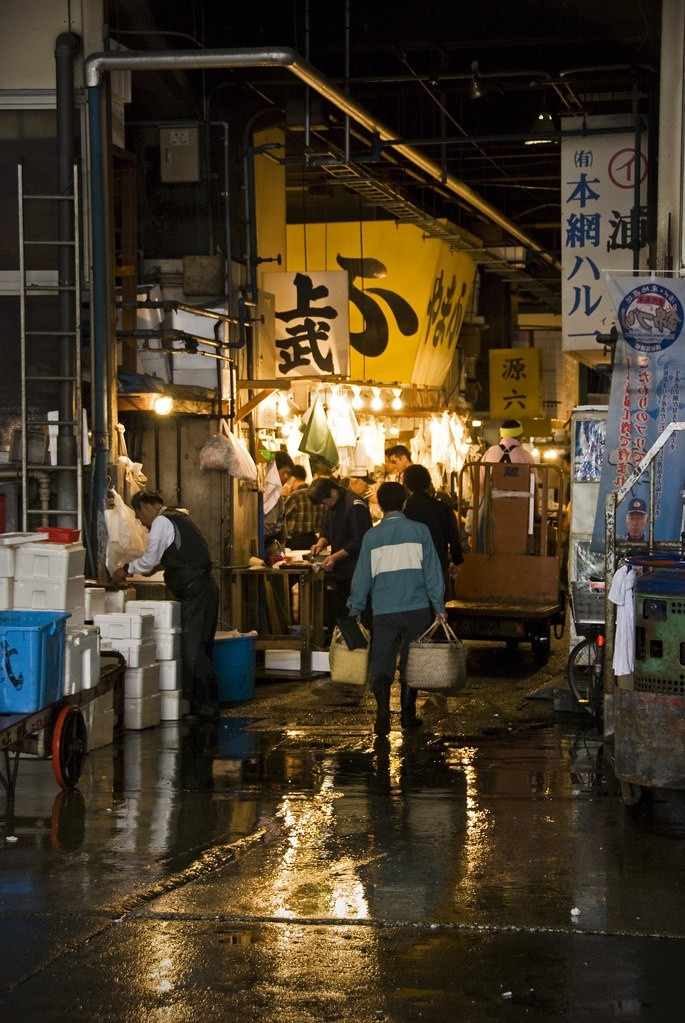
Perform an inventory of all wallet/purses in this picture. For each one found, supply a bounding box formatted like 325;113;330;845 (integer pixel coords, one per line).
335;615;368;651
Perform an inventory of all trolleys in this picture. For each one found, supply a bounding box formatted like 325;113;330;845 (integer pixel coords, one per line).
0;652;125;790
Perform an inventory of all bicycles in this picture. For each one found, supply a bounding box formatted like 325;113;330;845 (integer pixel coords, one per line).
569;579;606;736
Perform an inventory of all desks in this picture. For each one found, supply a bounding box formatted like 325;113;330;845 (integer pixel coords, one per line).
232;564;326;675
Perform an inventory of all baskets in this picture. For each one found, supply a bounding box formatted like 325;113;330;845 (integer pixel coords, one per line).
406;619;468;690
329;621;371;685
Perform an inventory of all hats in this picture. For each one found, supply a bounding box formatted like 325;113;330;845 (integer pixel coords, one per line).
499;420;524;439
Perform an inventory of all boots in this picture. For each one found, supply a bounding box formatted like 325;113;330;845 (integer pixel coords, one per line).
373;690;391;737
401;682;423;728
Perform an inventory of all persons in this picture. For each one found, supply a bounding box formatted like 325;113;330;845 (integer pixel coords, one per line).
471;417;540;554
625;498;649;542
347;481;449;734
260;446;464;646
113;489;220;720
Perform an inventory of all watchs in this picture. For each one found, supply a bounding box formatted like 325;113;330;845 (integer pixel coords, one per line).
123;564;133;577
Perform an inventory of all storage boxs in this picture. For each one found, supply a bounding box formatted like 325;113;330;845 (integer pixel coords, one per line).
0;532;183;761
311;649;332;671
266;648;301;671
